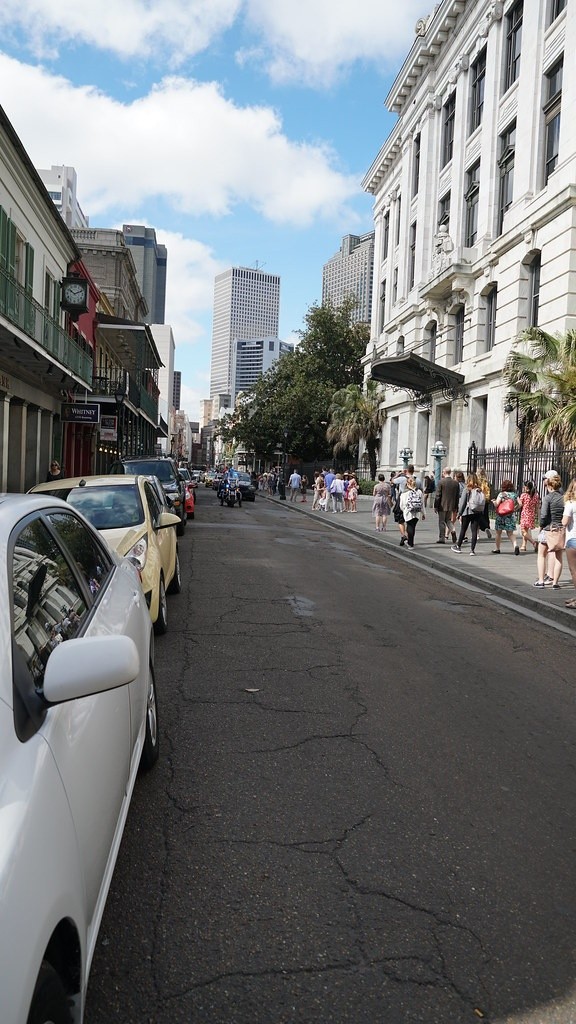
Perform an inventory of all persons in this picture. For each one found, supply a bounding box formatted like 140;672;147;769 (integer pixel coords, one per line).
251;471;280;495
533;470;565;590
90;565;103;595
312;467;359;513
434;466;492;555
214;466;228;474
32;612;80;707
219;467;242;507
288;470;308;502
561;478;576;608
47;460;63;482
491;480;540;555
372;464;435;549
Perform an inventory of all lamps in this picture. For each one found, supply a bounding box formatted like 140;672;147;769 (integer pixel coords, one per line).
72;383;80;392
398;446;413;458
431;440;447;455
61;374;67;385
98;442;122;457
46;363;53;376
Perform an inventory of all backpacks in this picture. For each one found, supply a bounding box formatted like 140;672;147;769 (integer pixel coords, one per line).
467;486;485;512
406;489;423;512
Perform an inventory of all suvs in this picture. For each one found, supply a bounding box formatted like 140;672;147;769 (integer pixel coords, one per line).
106;455;186;535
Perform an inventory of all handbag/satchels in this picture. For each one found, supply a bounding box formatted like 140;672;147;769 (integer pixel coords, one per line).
545;522;565;552
495;492;514;517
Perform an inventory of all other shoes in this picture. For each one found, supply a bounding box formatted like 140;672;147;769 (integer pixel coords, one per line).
312;507;357;513
400;535;407;545
435;527;492;556
532;573;560;589
515;546;519;555
520;547;526;551
565;597;576;609
492;549;500;554
407;543;414;550
533;541;538;553
374;527;386;532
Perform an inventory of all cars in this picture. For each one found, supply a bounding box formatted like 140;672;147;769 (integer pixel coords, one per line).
203;471;256;503
26;475;182;633
175;467;205;519
0;493;159;1024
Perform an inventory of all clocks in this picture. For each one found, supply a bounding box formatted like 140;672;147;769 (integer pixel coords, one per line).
60;270;90;321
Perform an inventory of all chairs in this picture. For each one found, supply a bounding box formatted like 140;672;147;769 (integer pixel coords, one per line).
93;375;110;392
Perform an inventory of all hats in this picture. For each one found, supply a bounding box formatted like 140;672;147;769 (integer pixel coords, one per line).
542;470;558;479
343;473;351;476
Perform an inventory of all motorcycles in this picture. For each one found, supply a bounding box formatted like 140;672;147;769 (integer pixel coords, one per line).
217;479;242;508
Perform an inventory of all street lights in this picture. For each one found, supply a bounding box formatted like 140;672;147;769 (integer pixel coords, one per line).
170;437;175;454
430;441;447;493
399;445;413;474
505;396;526;497
